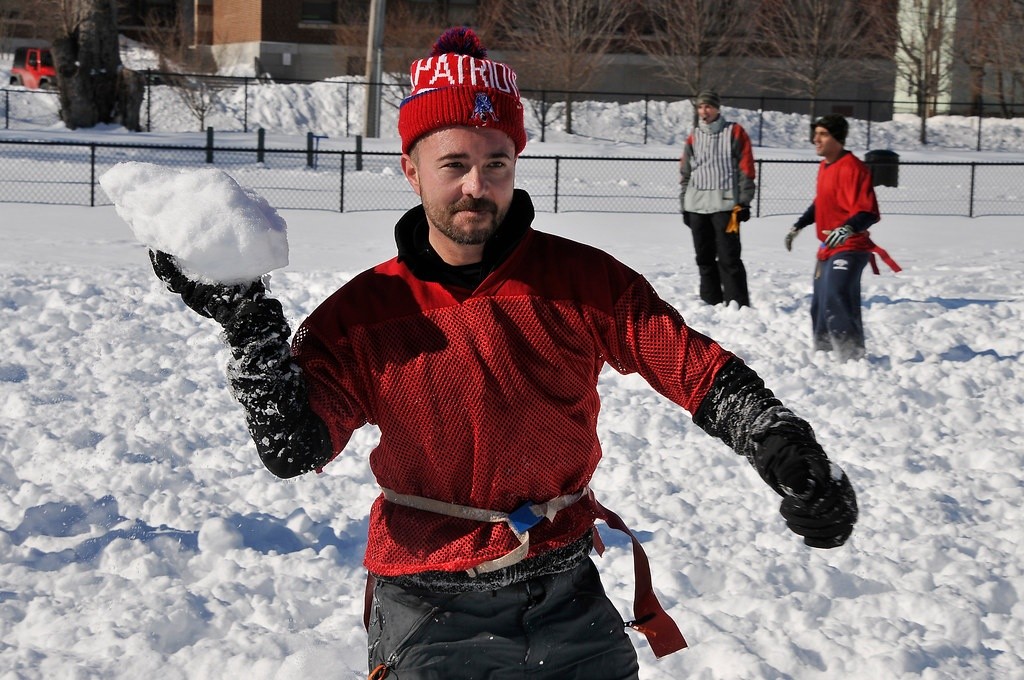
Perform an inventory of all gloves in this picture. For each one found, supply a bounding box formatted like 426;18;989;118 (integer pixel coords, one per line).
822;224;858;248
736;206;750;223
683;211;691;229
784;226;798;251
752;426;858;549
148;249;291;358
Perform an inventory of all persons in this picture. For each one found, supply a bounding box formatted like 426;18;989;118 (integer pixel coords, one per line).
679;90;756;309
785;115;903;368
149;26;860;680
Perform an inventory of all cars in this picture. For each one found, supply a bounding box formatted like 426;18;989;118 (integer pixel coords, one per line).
9;45;60;91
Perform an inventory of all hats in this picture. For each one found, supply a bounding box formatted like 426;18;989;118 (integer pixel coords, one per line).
811;114;849;145
400;27;526;159
696;89;720;109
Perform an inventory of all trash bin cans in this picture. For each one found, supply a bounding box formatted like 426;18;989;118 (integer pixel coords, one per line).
864;149;899;188
